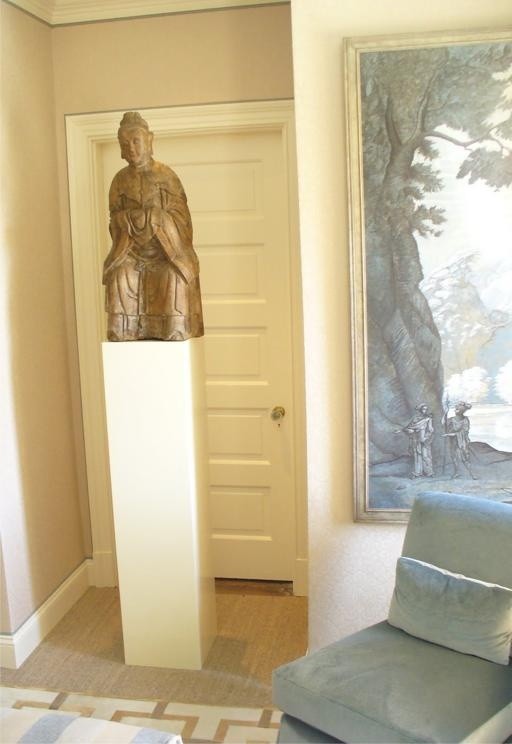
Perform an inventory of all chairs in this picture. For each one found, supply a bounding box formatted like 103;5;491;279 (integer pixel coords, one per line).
267;491;512;744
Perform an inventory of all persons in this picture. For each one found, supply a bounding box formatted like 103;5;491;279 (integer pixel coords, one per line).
391;402;436;480
98;111;204;341
441;402;479;481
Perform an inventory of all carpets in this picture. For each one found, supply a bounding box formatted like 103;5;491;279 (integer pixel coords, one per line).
0;682;282;744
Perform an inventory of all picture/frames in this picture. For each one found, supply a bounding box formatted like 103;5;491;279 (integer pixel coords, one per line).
339;26;511;527
